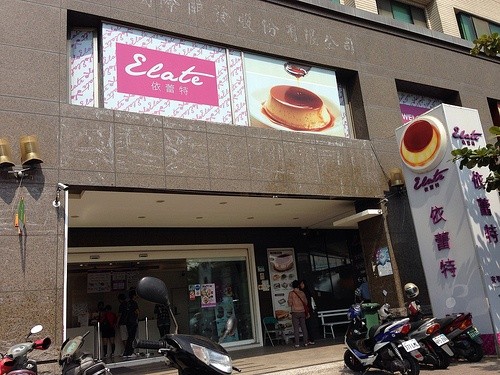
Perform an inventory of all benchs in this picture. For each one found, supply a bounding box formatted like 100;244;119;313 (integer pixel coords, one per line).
317;308;351;339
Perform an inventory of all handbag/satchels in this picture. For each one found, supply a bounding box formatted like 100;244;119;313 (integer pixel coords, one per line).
303;302;309;316
100;320;109;329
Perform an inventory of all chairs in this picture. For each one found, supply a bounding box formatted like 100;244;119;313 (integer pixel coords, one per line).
262;317;288;347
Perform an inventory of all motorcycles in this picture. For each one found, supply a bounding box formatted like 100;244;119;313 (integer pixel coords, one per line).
0;324;113;375
130;276;244;375
343;288;484;375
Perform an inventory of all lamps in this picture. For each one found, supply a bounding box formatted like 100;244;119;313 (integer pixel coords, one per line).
0;134;44;180
387;167;404;195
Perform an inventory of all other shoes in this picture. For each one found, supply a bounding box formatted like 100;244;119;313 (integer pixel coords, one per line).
103;355;107;360
128;352;138;357
305;344;308;346
123;352;129;357
294;344;300;347
109;353;114;358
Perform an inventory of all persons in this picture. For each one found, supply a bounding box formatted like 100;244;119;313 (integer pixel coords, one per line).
300;280;315;345
353;277;372;304
217;287;239;345
92;288;176;357
288;280;308;346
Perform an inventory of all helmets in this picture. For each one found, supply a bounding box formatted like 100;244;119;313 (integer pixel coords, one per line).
404;282;419;298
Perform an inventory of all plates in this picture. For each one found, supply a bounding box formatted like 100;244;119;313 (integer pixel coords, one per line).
249;86;345;137
272;253;294;274
